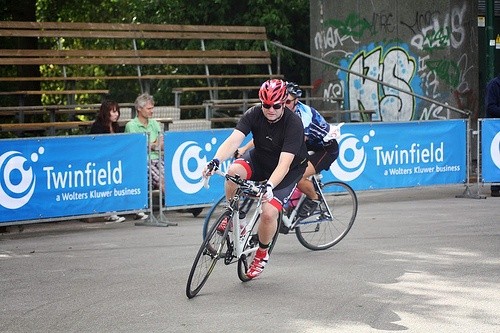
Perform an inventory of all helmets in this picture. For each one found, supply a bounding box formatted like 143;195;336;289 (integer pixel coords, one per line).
285;81;302;98
258;79;288;104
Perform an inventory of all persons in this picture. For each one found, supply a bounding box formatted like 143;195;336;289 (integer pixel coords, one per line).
90;100;149;224
202;79;308;277
232;83;339;218
124;92;204;217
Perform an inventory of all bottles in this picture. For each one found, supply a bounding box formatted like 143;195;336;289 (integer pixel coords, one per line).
239;210;247;235
288;187;302;207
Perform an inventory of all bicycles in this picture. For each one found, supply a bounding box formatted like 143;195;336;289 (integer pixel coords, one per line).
202;148;358;259
185;160;283;299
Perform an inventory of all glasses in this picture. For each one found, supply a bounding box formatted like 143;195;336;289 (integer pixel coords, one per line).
286;98;296;104
261;102;283;109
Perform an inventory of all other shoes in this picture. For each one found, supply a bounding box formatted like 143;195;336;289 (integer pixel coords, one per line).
192;207;205;217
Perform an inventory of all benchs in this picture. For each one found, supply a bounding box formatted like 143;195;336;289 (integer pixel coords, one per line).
0;85;376;138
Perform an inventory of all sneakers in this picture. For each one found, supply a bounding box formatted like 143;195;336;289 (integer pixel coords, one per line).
104;215;126;225
135;213;149;220
247;249;269;278
297;191;322;218
217;218;227;232
